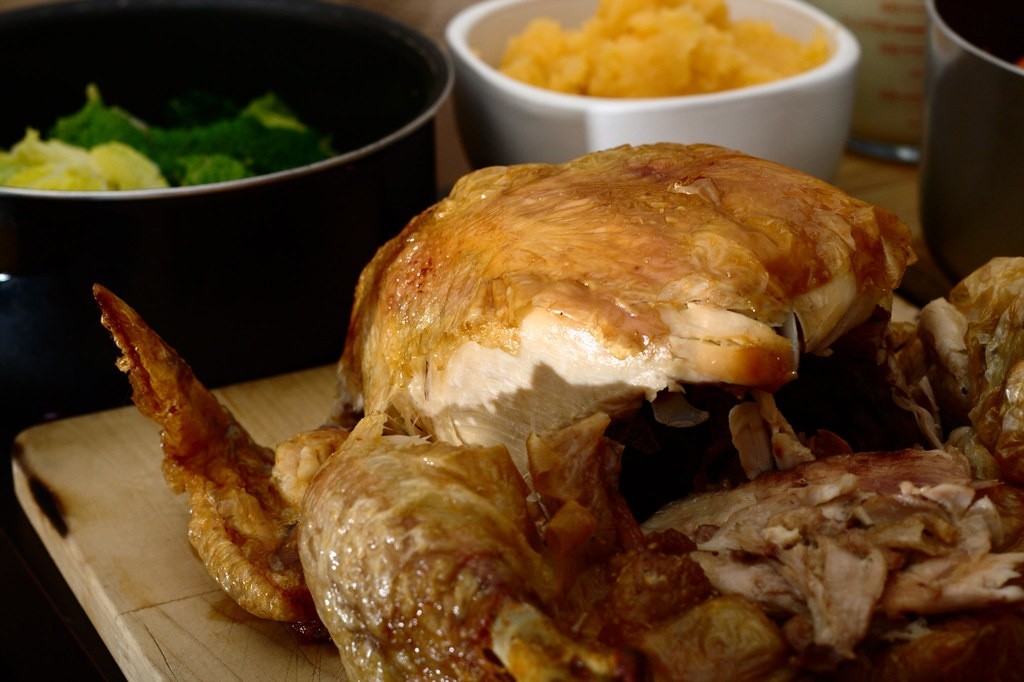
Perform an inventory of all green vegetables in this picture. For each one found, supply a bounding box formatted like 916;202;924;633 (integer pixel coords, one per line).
50;82;337;190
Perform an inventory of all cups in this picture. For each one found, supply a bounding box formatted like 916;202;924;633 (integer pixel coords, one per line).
918;0;1024;281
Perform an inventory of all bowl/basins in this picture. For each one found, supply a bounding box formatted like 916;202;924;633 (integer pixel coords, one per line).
445;0;862;187
0;0;455;395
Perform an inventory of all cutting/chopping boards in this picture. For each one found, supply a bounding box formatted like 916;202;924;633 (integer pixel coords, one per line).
11;360;356;682
